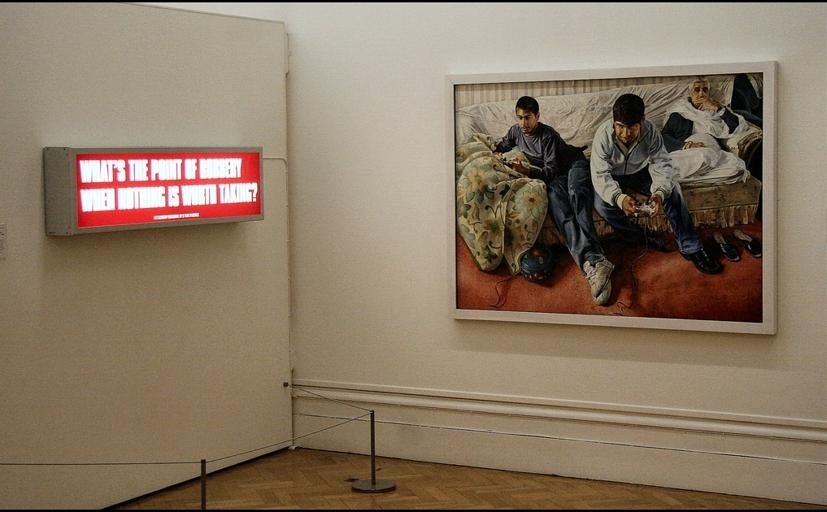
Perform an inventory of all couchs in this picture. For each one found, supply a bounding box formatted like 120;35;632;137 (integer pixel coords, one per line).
456;76;763;273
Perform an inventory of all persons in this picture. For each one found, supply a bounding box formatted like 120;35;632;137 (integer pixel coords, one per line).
493;96;616;306
589;94;723;273
662;76;746;186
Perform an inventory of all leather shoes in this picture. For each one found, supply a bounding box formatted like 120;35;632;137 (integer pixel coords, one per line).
680;247;723;275
647;236;666;250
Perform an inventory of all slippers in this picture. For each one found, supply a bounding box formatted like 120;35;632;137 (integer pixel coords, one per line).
732;228;762;259
712;230;741;261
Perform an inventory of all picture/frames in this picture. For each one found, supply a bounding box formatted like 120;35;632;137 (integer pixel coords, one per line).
444;61;777;338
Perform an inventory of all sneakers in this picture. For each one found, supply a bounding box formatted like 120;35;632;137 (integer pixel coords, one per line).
582;261;612;306
585;256;616;297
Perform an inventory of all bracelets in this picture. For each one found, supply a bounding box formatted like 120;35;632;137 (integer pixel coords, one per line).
682;142;693;150
